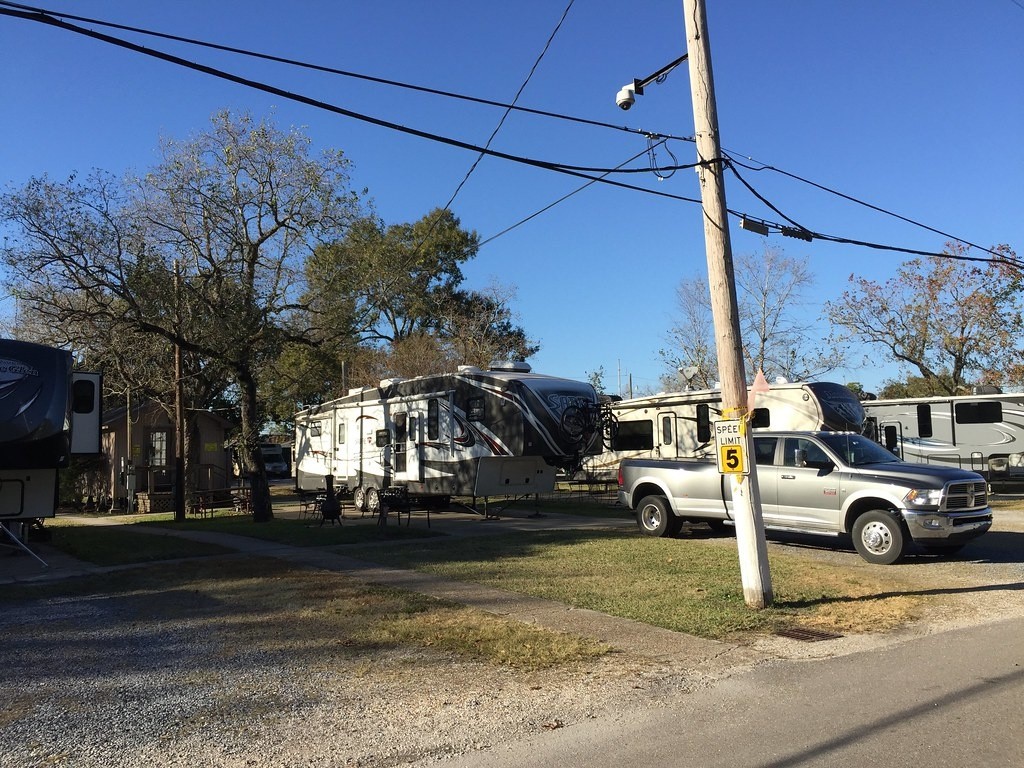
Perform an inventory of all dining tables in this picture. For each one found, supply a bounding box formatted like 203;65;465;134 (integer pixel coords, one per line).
377;489;450;527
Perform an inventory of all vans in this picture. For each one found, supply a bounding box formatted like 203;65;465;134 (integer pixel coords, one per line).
260;443;288;479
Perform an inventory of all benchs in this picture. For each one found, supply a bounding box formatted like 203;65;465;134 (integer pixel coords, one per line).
191;487;252;518
293;490;338;522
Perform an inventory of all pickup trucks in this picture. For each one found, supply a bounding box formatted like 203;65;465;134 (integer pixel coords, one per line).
617;429;994;566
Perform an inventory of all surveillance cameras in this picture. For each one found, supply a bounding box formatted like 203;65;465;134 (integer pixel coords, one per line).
615;90;635;111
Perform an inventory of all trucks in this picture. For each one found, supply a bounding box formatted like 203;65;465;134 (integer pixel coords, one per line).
859;385;1024;495
0;337;104;550
570;366;868;482
291;361;604;512
